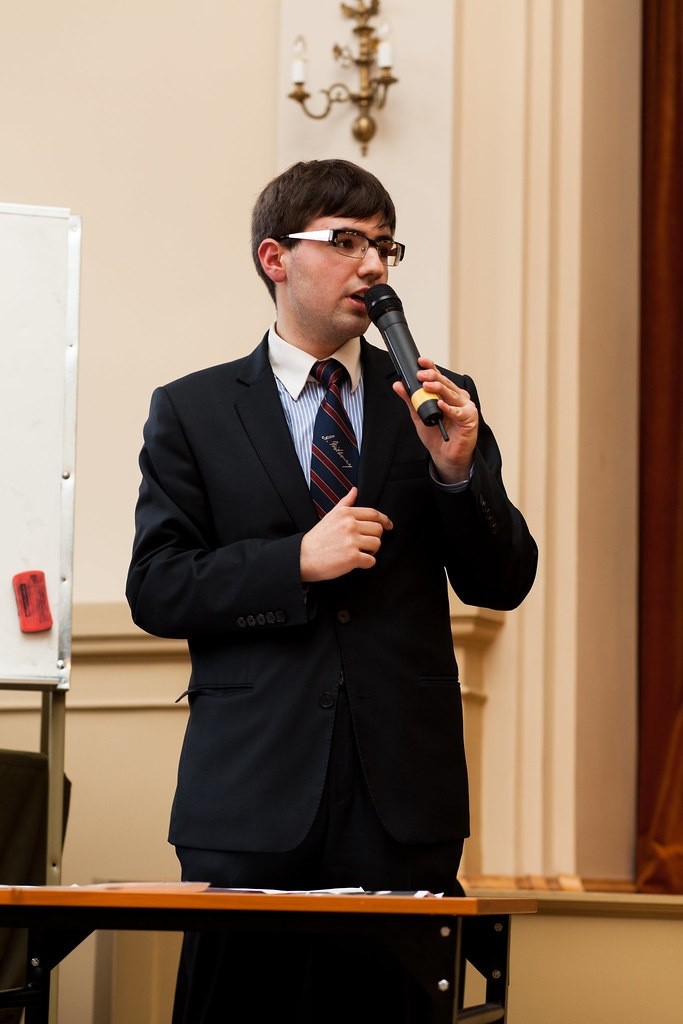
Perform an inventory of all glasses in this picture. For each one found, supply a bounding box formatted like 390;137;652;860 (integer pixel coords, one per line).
277;229;405;267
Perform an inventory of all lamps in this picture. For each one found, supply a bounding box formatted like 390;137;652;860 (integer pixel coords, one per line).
288;0;400;157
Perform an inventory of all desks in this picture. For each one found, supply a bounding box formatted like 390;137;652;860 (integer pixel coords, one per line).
0;885;537;1024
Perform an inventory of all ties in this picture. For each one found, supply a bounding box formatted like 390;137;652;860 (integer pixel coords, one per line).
309;358;360;520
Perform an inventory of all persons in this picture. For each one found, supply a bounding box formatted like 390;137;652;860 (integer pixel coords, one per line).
124;152;541;1023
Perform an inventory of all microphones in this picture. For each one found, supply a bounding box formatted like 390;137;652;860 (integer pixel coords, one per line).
363;284;449;441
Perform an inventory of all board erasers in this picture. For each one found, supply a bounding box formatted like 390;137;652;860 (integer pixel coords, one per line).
13;570;53;633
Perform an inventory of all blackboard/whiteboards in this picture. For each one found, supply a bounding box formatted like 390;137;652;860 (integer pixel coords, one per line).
1;202;82;690
